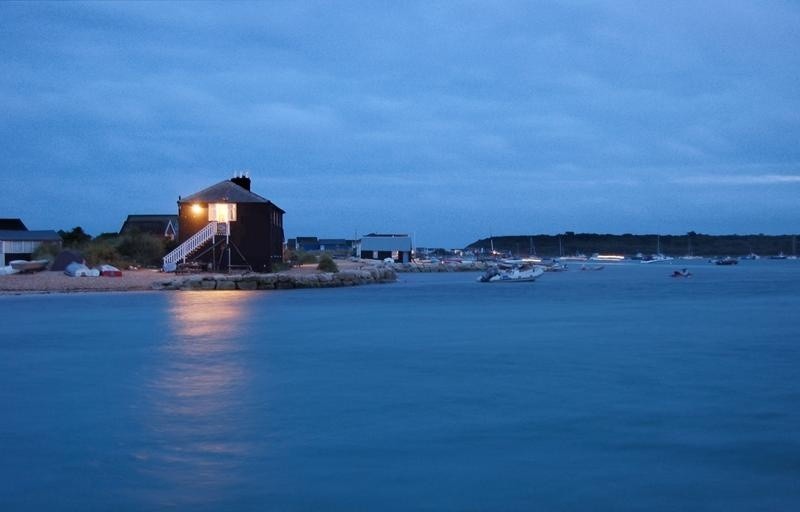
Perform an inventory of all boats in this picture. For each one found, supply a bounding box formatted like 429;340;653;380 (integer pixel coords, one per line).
8;258;49;271
